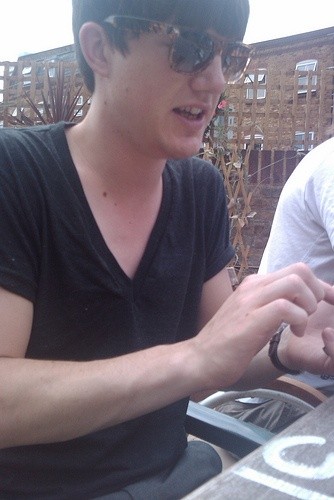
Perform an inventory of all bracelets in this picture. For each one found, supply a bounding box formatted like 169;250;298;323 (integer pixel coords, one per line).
269;325;305;376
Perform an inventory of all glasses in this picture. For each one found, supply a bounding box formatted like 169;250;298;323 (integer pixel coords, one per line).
103;15;257;84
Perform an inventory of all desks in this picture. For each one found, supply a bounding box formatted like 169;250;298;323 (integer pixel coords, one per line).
180;394;334;500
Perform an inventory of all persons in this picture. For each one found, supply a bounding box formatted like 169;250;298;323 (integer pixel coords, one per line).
205;136;334;463
1;1;334;500
